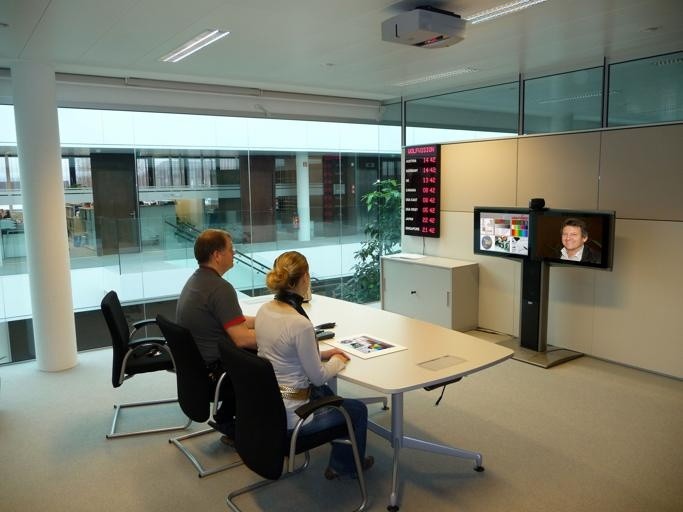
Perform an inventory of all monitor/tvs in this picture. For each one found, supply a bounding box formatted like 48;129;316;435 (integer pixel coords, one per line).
473;206;534;260
532;208;616;272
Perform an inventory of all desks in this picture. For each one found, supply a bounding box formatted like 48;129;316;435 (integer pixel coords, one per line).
238;292;516;510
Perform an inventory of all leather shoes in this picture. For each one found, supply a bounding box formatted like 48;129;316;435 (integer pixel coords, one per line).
325;456;374;480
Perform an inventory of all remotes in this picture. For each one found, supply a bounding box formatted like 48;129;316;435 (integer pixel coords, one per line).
316;332;335;340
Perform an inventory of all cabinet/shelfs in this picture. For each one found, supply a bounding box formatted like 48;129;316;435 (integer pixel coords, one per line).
380;253;478;332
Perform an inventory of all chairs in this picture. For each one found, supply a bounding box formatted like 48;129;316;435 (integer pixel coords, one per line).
215;340;367;511
153;312;260;479
99;290;194;439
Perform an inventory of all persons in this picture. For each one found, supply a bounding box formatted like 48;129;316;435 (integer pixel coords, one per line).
254;251;374;480
554;218;598;265
176;229;257;448
0;209;11;219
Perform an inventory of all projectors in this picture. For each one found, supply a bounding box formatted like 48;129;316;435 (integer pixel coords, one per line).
381;5;467;50
